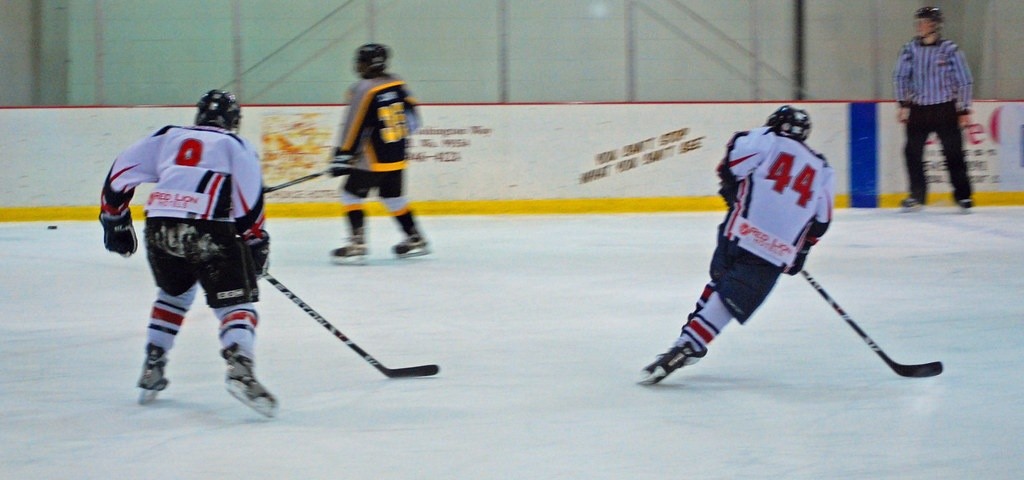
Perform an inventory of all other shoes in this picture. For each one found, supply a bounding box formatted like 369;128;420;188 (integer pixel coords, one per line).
330;228;367;265
901;197;925;213
956;199;974;214
391;223;429;259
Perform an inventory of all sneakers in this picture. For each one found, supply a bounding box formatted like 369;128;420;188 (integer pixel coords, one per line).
225;356;276;418
635;338;707;386
136;343;167;404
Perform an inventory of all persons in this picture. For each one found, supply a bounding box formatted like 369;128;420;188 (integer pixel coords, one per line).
644;106;832;384
329;44;421;257
99;89;276;405
892;6;974;208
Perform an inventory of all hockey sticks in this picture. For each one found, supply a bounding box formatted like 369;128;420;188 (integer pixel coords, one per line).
262;167;334;194
261;271;440;380
803;269;946;379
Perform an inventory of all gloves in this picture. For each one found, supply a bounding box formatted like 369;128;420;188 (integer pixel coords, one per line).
245;229;269;274
101;208;138;254
326;146;356;177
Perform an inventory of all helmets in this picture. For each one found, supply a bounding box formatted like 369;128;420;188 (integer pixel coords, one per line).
914;7;945;23
356;44;387;73
195;89;241;130
766;105;812;141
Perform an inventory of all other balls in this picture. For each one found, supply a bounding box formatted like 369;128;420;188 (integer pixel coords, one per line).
47;225;58;229
